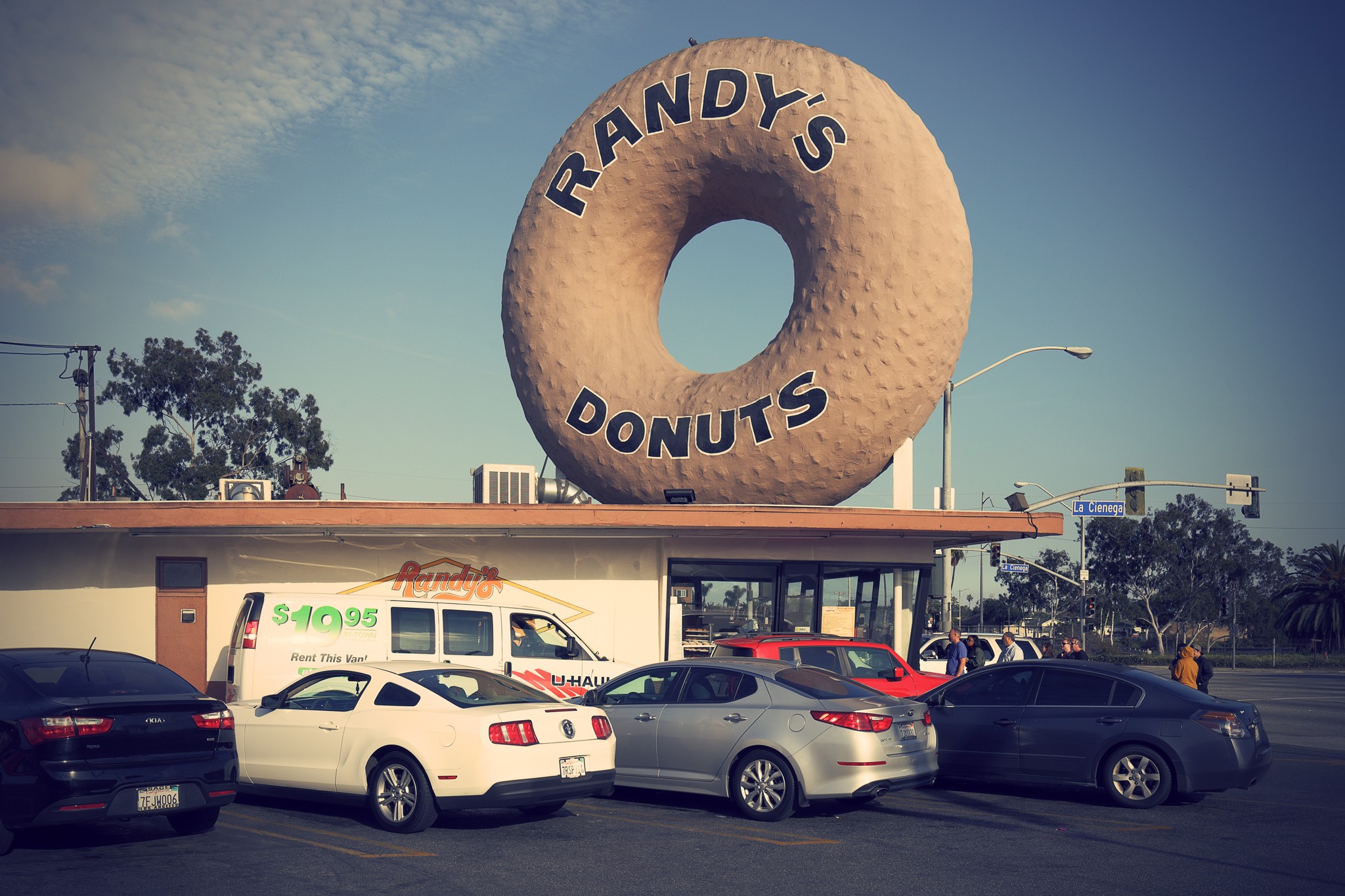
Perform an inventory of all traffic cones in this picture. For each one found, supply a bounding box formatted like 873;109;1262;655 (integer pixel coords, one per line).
1324;651;1328;663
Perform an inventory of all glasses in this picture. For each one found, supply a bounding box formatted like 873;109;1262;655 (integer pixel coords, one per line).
949;633;958;635
966;639;974;642
1062;642;1070;645
1071;642;1075;645
1002;636;1009;639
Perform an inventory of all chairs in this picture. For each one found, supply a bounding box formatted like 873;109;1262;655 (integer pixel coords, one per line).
692;681;713;702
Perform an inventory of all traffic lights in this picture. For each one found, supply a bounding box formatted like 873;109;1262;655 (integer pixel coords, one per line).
1221;595;1232;618
1088;623;1094;630
1084;594;1096;618
990;542;1000;567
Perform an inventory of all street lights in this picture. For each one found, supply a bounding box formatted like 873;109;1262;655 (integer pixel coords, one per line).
941;345;1094;648
1013;481;1086;652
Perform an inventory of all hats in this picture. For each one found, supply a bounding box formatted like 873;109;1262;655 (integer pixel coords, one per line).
1190;644;1204;655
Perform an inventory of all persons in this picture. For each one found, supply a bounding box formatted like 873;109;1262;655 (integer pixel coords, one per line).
996;632;1024;663
1056;637;1075;659
1069;637;1088;661
1168;642;1213;694
944;629;967;678
963;635;985;673
1041;642;1056;659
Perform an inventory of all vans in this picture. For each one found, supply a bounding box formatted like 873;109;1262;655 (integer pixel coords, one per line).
225;593;652;704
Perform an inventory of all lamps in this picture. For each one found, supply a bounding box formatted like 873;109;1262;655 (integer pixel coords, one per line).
663;489;696;504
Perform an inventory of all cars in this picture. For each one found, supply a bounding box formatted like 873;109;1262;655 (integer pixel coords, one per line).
1;647;240;849
900;657;1273;808
224;660;616;834
564;656;939;822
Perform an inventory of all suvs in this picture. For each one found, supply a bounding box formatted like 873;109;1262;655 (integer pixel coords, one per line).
709;632;956;698
681;609;795;640
919;632;1042;675
